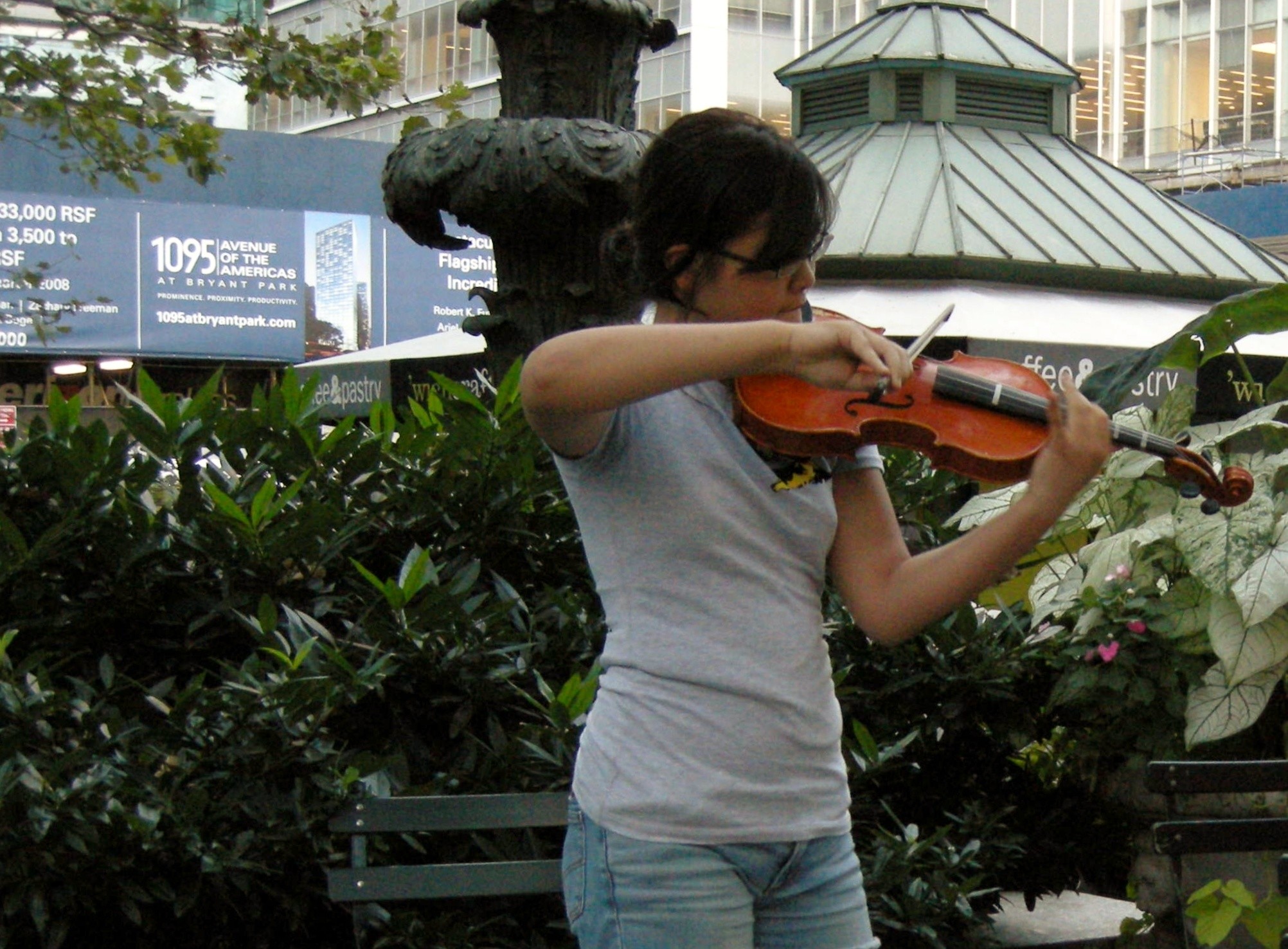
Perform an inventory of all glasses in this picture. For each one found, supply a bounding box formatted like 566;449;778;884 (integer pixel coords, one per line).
705;232;834;280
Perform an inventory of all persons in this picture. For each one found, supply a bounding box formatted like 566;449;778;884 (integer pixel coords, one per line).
519;106;1112;948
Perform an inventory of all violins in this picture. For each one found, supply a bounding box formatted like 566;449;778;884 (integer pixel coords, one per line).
729;304;1255;514
